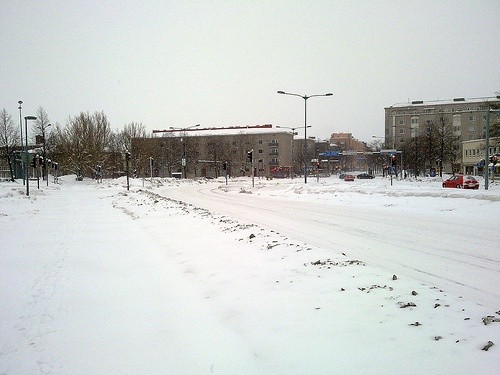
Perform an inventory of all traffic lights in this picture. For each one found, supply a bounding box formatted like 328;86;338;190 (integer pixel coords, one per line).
489;156;493;163
223;162;227;171
247;151;253;163
126;152;131;161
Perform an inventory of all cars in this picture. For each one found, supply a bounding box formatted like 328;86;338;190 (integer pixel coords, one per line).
344;174;355;181
357;173;375;179
442;175;479;190
339;173;355;179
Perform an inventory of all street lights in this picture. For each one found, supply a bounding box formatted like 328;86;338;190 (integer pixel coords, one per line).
36;123;52;181
371;135;395;186
17;100;25;185
275;126;312;179
24;115;37;196
276;90;334;184
169;124;200;179
